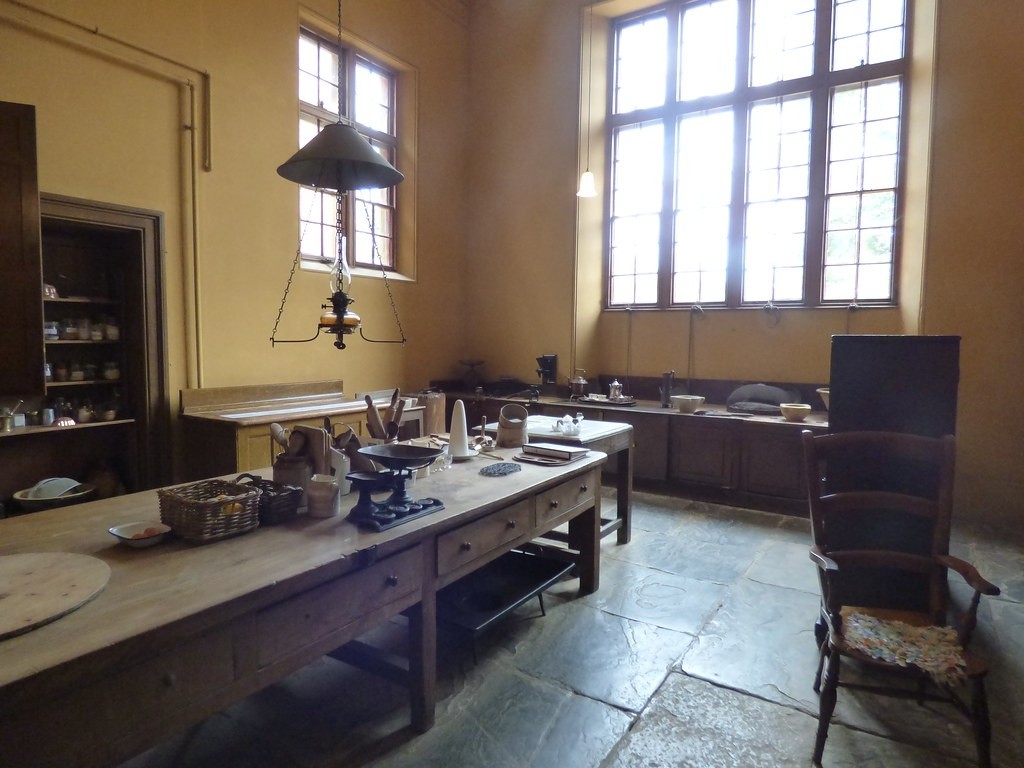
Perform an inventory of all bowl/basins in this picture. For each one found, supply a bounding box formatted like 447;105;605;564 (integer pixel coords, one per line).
13;478;96;513
108;520;172;548
780;403;811;421
671;395;706;413
817;388;829;410
54;417;75;427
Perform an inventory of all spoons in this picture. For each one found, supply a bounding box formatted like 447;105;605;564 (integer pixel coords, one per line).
387;421;399;439
289;431;306;456
270;423;289;456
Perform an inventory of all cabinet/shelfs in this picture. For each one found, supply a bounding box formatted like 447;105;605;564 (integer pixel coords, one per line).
1;192;159;518
182;396;394;482
433;385;830;521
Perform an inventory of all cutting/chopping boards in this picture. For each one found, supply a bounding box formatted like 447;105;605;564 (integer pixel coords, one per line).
0;553;111;641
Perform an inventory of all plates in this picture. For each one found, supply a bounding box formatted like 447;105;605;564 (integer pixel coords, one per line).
445;449;480;460
357;444;444;470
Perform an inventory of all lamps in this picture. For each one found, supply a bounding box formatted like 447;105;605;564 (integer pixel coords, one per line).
270;0;409;349
576;0;598;199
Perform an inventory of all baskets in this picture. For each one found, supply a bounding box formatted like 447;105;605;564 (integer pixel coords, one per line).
156;479;263;541
230;473;304;522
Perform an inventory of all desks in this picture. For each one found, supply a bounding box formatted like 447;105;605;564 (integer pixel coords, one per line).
355;397;427;442
471;414;635;577
0;432;609;768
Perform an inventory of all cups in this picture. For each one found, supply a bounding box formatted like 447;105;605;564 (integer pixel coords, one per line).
598;395;606;400
589;394;597;399
308;475;340;517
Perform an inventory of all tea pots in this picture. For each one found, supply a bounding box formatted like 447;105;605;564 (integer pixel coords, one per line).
609;379;623;400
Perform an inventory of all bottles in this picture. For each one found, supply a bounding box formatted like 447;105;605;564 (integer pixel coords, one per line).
275;453;312;510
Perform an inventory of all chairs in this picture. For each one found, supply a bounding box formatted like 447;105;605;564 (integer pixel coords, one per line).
802;429;1001;768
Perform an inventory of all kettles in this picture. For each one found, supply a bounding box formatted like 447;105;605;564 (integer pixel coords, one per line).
564;368;588;402
